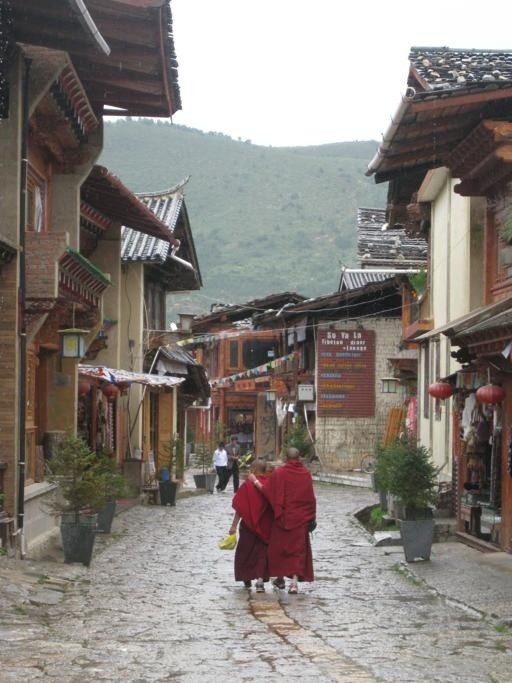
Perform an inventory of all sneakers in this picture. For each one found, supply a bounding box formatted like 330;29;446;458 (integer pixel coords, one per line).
273;578;285;589
256;582;265;593
245;579;252;587
288;583;298;594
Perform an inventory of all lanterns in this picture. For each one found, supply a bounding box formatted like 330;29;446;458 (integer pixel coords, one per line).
77;380;91;401
475;383;506;408
103;381;120;400
427;381;454;406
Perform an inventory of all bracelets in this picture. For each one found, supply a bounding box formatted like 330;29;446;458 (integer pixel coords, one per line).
253;479;259;486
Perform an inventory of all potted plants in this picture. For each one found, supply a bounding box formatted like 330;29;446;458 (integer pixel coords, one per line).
159;432;189;506
190;433;217;489
374;419;440;563
41;424;133;566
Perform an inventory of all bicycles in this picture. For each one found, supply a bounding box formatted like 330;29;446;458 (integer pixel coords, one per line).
359;453;379;475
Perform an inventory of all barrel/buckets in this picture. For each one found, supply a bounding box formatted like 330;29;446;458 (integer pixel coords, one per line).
43;430;67;475
159;470;169;481
35;444;45;483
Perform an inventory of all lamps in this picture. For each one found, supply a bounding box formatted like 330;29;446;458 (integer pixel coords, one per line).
57;305;91;358
266;377;277;400
380;363;400;393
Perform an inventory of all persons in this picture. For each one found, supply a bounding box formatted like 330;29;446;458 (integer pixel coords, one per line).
215;435;241;493
230;458;275;593
212;441;229;487
247;447;318;592
